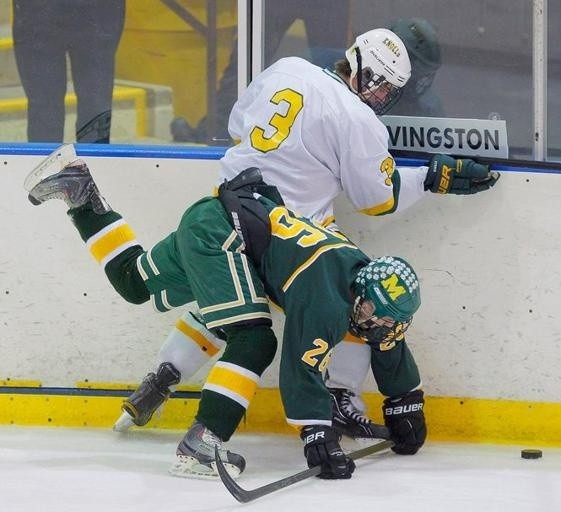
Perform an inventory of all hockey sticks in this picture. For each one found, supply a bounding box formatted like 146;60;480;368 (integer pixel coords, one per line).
215;439;393;503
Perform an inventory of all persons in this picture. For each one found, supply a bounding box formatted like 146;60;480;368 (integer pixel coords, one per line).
121;26;504;441
171;1;351;146
316;16;450;139
11;1;128;144
26;157;429;481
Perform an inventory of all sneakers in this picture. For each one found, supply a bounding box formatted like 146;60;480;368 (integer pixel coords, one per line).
27;158;113;215
324;387;393;441
175;419;246;475
121;362;182;426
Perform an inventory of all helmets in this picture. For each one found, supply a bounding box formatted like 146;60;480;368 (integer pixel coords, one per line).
351;254;422;346
389;15;442;103
344;27;412;118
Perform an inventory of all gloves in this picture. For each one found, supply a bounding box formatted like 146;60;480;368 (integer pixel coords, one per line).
382;389;427;455
423;153;501;196
300;424;356;479
